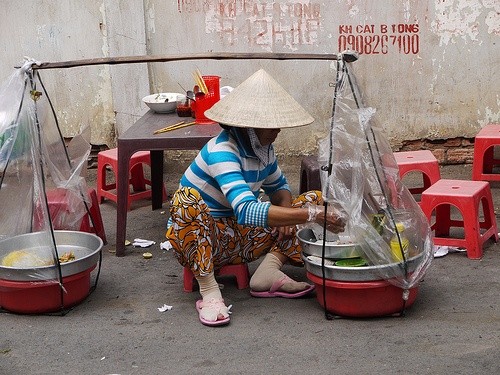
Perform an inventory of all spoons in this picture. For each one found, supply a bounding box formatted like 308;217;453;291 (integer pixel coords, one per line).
193;85;200;94
186;90;196;100
195;92;205;99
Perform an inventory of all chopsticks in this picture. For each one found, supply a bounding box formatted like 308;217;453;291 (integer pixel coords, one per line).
190;65;208;94
152;120;196;134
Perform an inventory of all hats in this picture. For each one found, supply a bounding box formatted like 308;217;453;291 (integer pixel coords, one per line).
204;68;315;128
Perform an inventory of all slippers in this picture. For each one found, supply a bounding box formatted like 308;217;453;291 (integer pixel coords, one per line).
250;275;315;297
196;299;230;325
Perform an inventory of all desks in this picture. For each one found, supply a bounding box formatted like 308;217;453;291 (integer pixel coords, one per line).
115;109;225;256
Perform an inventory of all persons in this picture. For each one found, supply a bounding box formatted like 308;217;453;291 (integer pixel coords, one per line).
166;68;347;326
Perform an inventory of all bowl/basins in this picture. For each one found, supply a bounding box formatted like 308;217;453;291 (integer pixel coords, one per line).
142;92;184;114
0;264;96;314
305;271;418;318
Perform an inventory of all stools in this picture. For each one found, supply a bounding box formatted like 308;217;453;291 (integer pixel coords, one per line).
421;178;497;259
299;155;322;193
98;149;167;212
182;263;249;294
471;125;500;183
384;150;441;210
34;188;108;246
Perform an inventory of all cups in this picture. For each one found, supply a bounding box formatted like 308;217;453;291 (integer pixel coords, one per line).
177;95;191;117
